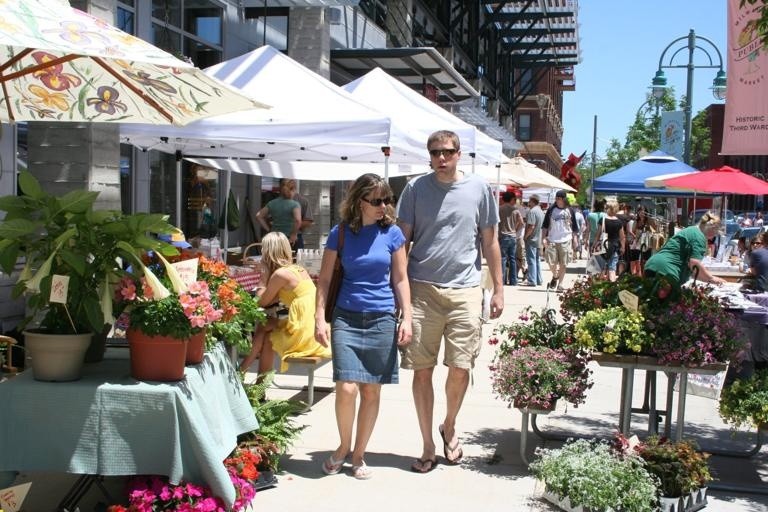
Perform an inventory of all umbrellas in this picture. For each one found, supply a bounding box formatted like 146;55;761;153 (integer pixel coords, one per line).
643;172;711;226
662;165;767;235
484;155;577;193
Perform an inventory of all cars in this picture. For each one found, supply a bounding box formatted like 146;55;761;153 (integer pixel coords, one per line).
731;226;768;241
739;212;768;225
688;209;742;237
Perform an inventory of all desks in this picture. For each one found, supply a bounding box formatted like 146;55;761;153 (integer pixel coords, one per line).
595;354;727;446
2;341;224;512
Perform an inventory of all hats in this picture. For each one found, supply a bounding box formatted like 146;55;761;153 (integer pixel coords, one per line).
556;191;566;197
530;194;540;200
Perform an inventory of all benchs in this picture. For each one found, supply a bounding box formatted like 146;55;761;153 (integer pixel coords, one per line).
512;398;556;469
284;356;332;410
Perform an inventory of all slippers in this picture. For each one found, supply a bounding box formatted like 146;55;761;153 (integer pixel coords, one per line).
439;424;462;463
412;458;439;473
352;466;371;479
322;455;344;474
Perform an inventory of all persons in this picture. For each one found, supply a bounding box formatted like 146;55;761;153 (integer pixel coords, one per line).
255;180;304;262
238;232;332;374
197;196;214;237
278;177;314;252
394;130;506;475
312;172;414;480
492;188;767;311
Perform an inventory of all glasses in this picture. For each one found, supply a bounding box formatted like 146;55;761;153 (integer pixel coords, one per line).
361;197;390;206
751;242;760;245
429;150;457;157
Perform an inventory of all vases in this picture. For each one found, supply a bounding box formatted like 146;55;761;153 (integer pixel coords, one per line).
126;324;206;383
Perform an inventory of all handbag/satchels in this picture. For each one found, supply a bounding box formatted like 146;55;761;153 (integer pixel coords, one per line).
632;227;649;252
218;190;240;231
591;217;608;256
325;224;344;322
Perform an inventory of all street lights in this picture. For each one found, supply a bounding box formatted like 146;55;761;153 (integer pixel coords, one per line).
650;30;727;225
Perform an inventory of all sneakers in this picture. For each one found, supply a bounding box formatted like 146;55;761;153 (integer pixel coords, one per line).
503;271;563;292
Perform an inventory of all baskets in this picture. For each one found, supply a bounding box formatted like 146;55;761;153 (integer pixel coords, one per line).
243;243;262;273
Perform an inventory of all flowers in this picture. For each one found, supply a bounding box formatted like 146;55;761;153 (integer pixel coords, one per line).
551;269;754;373
527;429;720;512
111;245;267;354
490;299;595;415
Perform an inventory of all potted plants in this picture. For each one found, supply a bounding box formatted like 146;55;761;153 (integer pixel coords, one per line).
0;170;184;382
716;363;767;438
